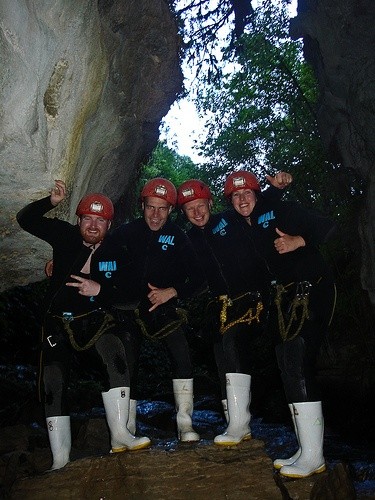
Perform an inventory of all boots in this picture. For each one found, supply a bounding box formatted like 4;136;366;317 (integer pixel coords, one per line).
222;399;230;425
172;378;200;442
214;373;252;446
280;401;325;478
45;416;71;472
102;386;151;453
273;404;302;470
126;399;137;436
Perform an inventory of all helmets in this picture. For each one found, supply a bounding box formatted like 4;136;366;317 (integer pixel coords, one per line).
140;178;178;206
224;171;259;200
177;179;211;208
75;193;115;220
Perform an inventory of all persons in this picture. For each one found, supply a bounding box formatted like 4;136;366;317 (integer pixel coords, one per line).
225;170;332;478
175;170;294;446
16;180;152;474
46;178;202;454
